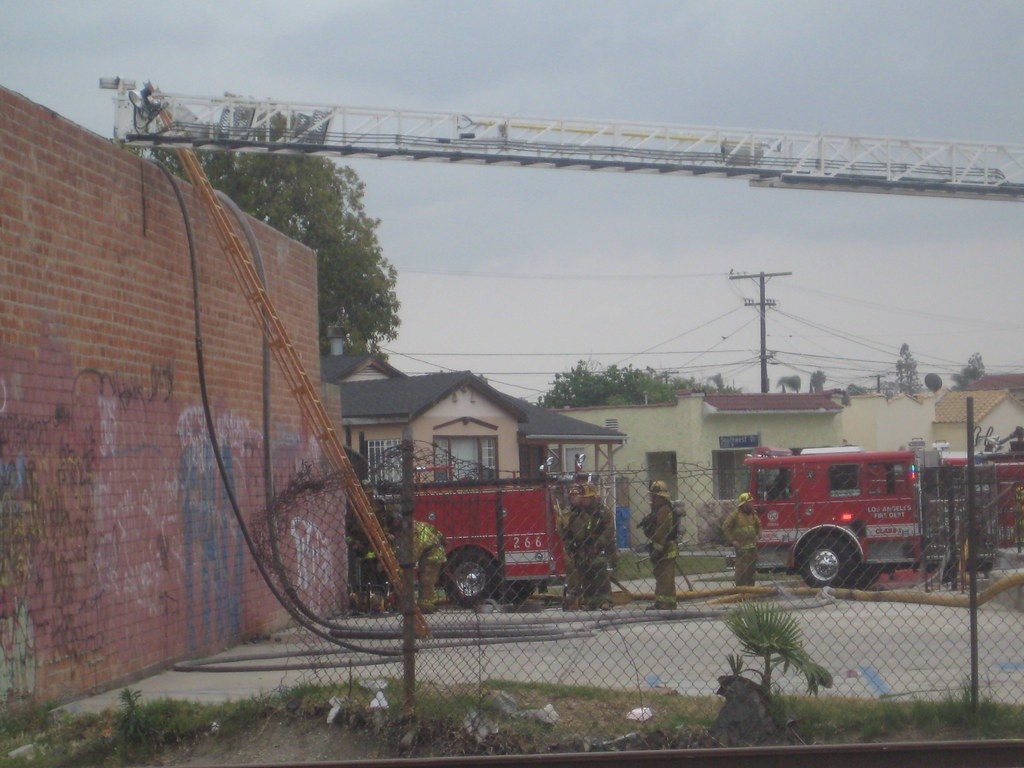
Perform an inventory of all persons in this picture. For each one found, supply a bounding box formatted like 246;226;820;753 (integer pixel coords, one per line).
347;480;447;615
557;483;617;612
722;493;762;585
647;481;678;609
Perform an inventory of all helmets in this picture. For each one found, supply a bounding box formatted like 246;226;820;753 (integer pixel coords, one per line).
581;485;596;497
738;492;753;506
647;481;670;497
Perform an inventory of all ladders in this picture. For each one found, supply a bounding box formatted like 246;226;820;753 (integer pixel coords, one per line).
147;81;431;639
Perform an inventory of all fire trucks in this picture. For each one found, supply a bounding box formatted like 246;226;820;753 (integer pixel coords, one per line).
743;423;1024;589
354;478;568;609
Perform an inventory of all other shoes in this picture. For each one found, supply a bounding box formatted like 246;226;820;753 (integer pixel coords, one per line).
602;601;610;610
646;604;657;610
581;603;593;611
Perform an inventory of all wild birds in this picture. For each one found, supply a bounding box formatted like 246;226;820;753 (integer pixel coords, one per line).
721;336;728;340
